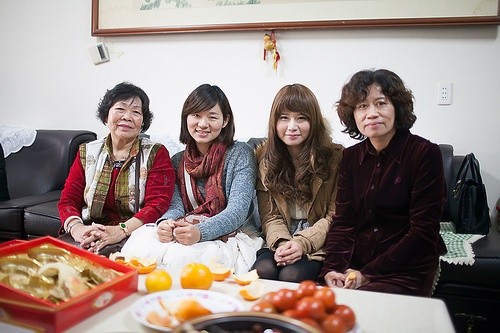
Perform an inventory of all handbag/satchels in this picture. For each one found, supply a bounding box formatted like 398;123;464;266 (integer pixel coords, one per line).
58;232;130;255
172;214;237;243
442;152;490;235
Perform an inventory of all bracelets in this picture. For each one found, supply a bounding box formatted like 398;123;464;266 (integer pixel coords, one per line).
119;222;129;237
66;219;81;234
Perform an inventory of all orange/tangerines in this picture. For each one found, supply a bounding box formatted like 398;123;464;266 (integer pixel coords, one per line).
180;263;212;290
209;264;231;282
239;284;261;300
234;269;258;285
145;269;172;293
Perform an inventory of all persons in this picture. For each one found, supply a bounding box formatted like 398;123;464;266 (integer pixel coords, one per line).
121;84;266;277
318;70;448;296
57;82;176;258
248;84;345;283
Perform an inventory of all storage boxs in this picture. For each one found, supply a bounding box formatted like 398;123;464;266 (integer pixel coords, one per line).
0;235;139;333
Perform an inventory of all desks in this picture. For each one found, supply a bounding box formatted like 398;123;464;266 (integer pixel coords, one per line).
0;276;457;333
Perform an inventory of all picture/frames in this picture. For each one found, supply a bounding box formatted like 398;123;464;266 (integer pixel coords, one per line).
91;0;500;38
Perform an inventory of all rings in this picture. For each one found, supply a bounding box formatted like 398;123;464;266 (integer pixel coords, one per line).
99;239;103;243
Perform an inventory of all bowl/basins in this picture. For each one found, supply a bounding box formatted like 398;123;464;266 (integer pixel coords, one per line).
168;311;318;333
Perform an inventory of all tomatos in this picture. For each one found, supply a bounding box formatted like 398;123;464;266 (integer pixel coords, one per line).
248;280;355;333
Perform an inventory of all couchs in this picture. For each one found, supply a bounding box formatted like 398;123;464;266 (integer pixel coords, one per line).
0;131;500;333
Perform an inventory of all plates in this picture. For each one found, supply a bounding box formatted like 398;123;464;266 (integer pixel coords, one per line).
250;301;363;332
128;288;248;331
